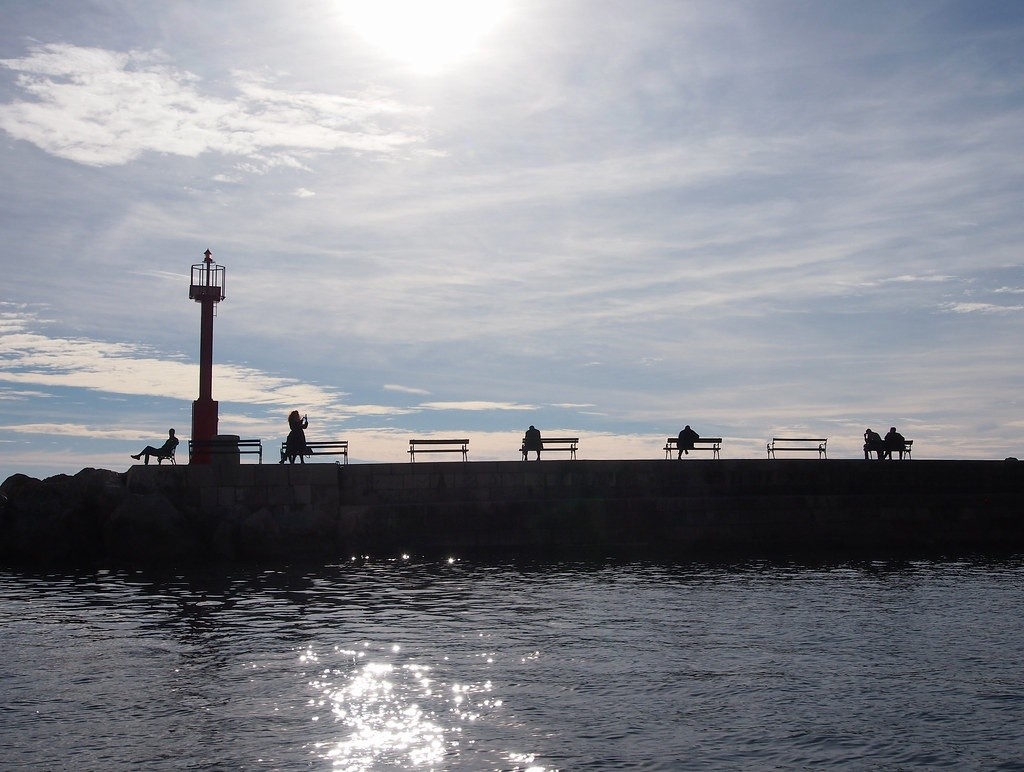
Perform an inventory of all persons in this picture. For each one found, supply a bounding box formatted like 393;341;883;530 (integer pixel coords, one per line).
279;411;313;463
863;427;905;459
522;425;544;460
677;425;699;460
131;428;180;464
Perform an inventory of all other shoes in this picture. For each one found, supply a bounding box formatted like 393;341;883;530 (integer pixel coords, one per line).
130;455;140;460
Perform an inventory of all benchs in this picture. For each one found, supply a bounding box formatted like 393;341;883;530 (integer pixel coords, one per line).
865;440;914;460
663;438;722;459
767;438;829;459
188;439;262;465
280;440;348;465
407;439;469;463
157;442;179;465
519;438;579;461
